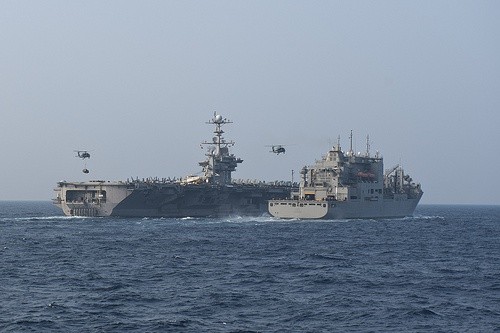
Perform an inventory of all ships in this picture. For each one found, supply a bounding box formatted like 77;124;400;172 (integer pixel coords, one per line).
268;129;423;218
53;112;300;220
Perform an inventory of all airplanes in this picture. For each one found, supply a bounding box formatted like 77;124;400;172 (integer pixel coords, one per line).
74;149;90;159
265;143;295;155
